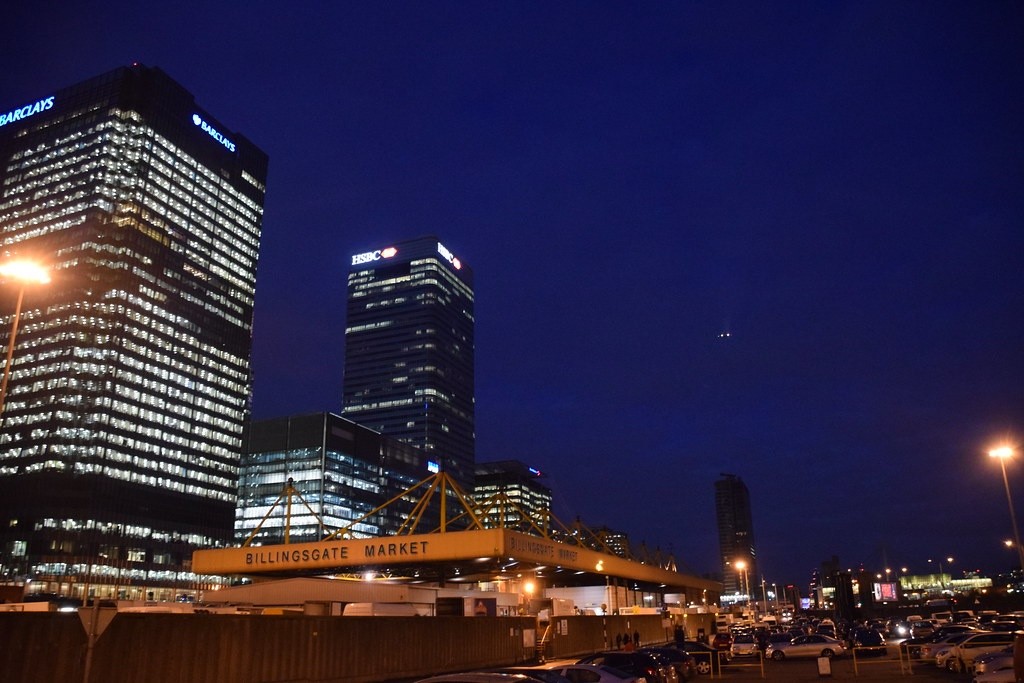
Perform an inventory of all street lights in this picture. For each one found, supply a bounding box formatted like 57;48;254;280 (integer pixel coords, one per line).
734;562;751;608
990;446;1023;569
0;259;50;408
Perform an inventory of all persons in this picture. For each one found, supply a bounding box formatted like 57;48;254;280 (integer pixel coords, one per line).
616;633;622;650
633;630;639;647
623;633;629;645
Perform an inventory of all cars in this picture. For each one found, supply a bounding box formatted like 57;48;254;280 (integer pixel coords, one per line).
415;639;731;683
712;610;1024;683
